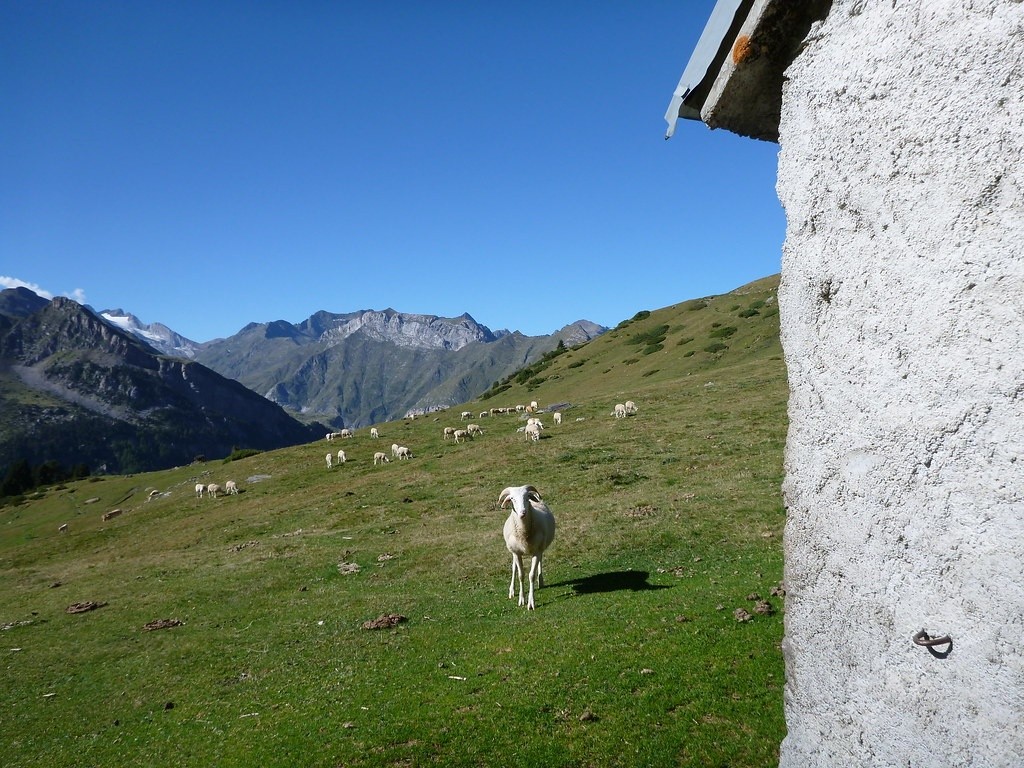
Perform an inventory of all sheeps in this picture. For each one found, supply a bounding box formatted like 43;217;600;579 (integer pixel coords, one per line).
370;426;380;438
326;429;353;440
326;453;333;467
373;451;389;466
102;509;122;522
337;450;346;464
498;485;556;611
195;480;240;498
392;444;413;460
610;400;638;419
444;400;562;442
58;523;68;532
